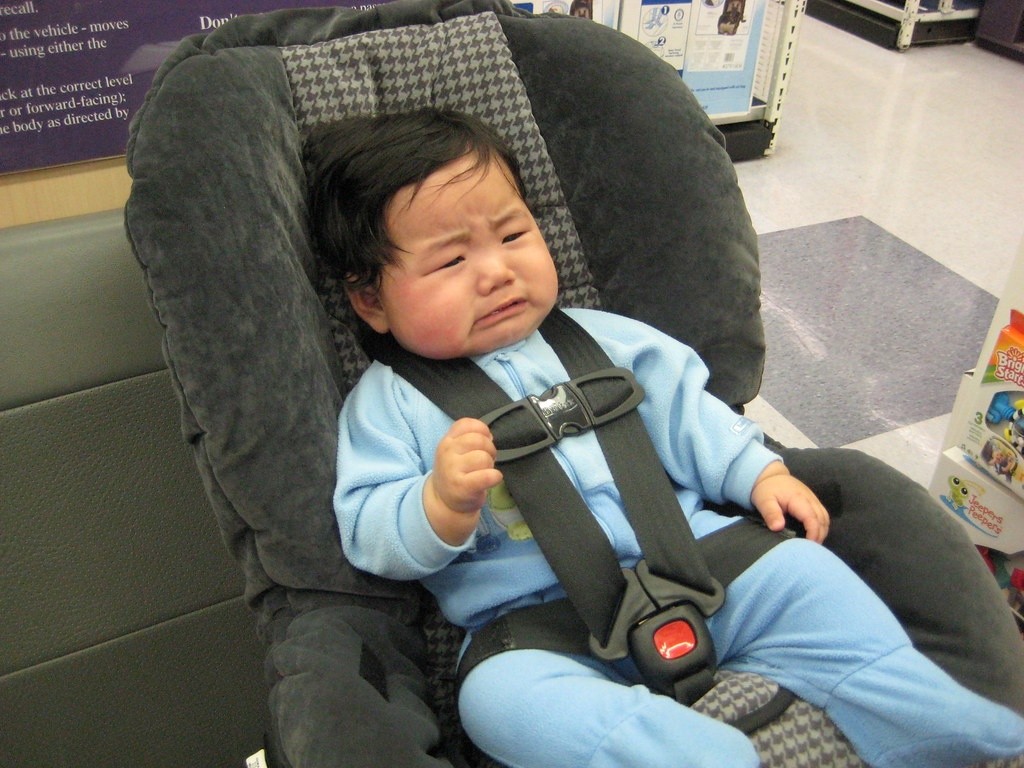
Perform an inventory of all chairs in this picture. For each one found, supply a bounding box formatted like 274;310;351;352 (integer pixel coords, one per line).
120;0;1024;768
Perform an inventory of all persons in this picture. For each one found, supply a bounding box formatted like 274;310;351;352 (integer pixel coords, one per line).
295;108;1024;768
